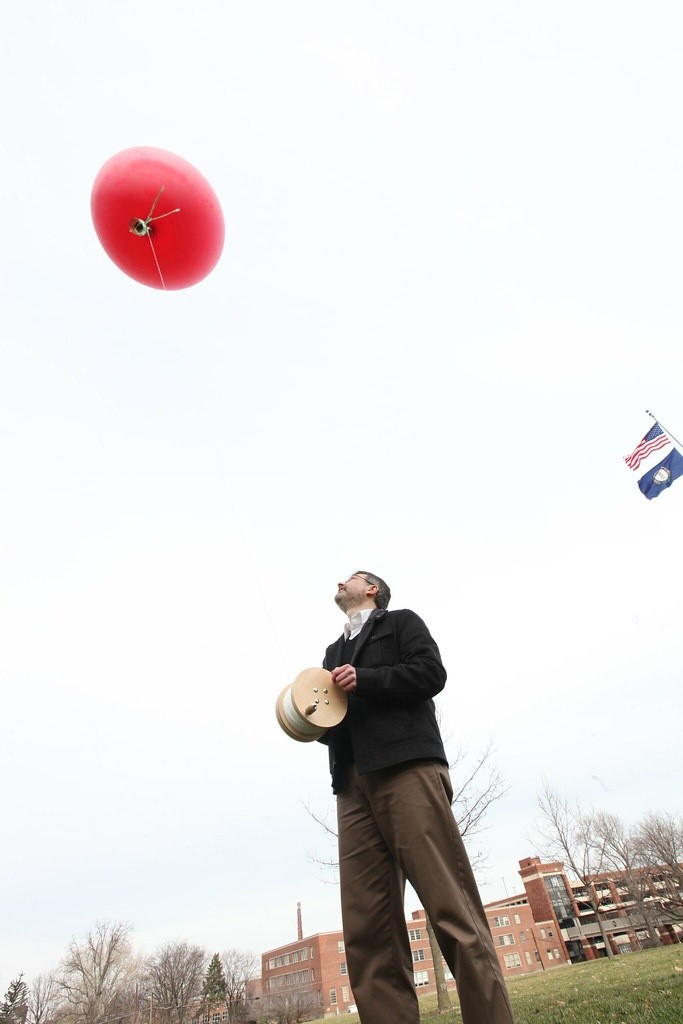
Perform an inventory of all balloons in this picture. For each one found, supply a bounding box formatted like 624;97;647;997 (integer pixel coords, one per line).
91;147;226;291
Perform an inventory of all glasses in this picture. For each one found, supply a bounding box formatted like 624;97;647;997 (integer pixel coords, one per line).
345;573;379;593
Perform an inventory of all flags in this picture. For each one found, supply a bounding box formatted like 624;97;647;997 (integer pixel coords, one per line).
622;422;683;499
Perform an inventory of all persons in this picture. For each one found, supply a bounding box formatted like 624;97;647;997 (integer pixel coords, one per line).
321;570;514;1024
